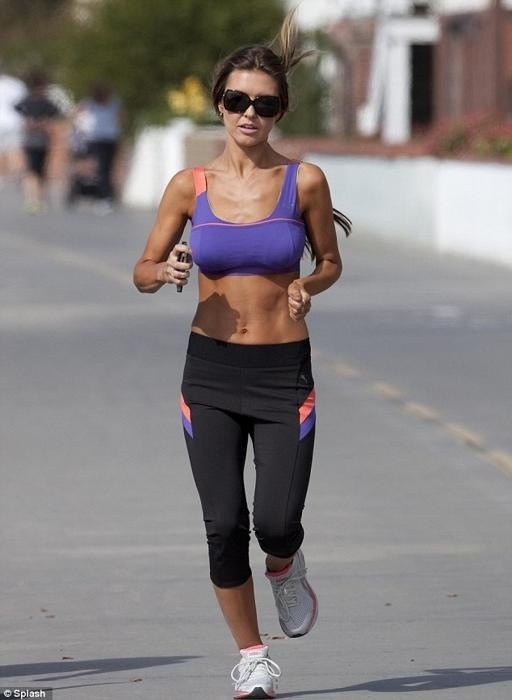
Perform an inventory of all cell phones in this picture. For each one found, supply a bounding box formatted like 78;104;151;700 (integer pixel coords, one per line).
177;242;186;292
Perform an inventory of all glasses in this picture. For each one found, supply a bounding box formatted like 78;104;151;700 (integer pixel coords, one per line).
220;89;281;117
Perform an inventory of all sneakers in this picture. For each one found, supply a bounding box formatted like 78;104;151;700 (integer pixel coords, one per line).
231;645;282;699
265;548;319;639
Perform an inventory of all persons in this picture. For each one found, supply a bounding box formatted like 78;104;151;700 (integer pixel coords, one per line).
132;0;352;697
68;80;125;216
13;67;68;214
